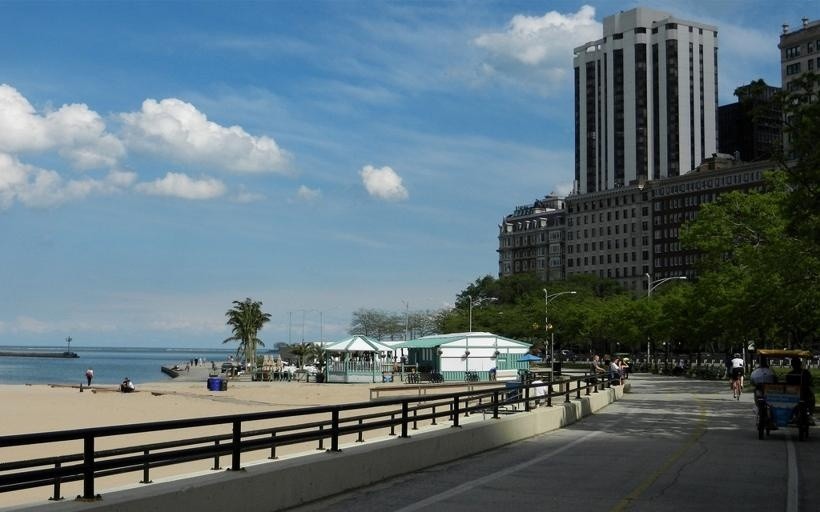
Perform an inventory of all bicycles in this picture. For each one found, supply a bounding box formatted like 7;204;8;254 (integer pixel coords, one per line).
735;372;743;401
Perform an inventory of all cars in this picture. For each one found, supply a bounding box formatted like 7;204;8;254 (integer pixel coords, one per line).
221;357;245;374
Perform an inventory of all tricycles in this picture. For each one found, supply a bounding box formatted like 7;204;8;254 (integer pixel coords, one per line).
755;350;812;441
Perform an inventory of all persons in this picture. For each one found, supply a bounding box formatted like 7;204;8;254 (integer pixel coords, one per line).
731;352;746;398
785;357;815;419
227;354;233;363
127;380;135;392
187;355;205;372
488;367;498;381
121;378;129;392
211;361;217;371
86;367;94;386
585;354;629;391
546;351;551;364
750;358;776;408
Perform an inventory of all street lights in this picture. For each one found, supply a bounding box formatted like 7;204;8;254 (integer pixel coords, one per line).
466;295;497;333
645;272;687;366
289;308;324;347
542;289;579;357
403;297;407;341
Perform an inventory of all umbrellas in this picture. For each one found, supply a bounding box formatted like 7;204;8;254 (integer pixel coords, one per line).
518;353;541;371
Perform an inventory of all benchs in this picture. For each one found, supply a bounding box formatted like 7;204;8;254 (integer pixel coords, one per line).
369;378;510;401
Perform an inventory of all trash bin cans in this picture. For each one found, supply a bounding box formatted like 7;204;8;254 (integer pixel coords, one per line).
208;376;221;391
